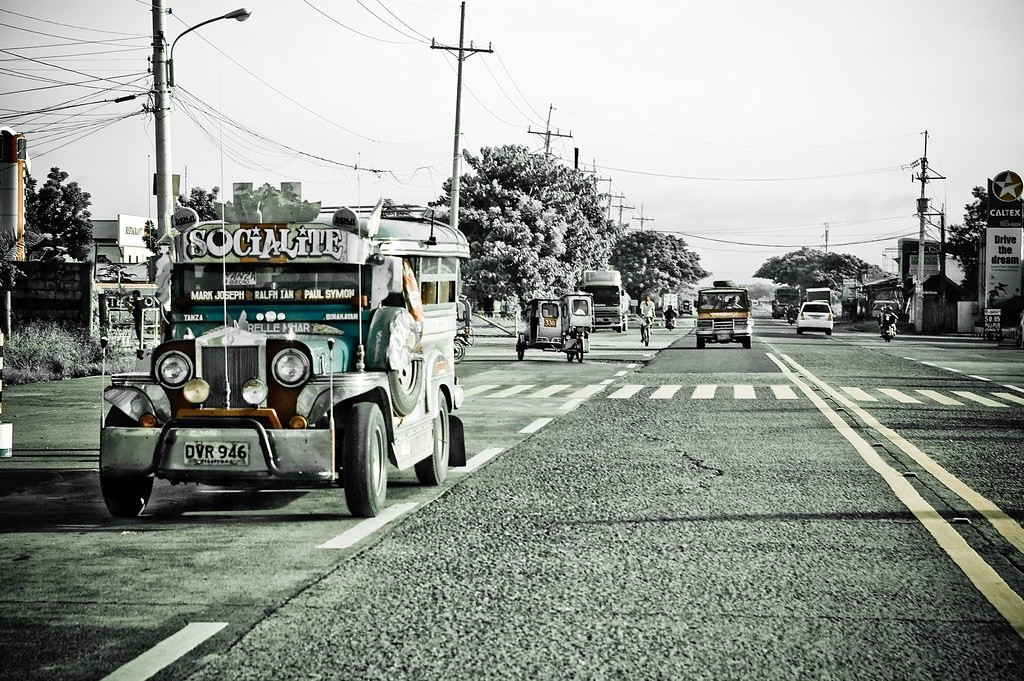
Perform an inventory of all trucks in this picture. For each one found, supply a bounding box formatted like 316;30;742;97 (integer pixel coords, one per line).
806;287;832;311
578;270;632;334
693;281;754;349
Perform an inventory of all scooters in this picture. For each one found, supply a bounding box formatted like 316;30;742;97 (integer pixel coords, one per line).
884;324;896;343
665;317;676;332
789;316;794;326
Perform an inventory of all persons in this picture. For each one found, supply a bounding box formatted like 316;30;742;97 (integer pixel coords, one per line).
877;304;899;337
572;302;584;315
126;290;146;349
700;295;799;321
639;295;676;344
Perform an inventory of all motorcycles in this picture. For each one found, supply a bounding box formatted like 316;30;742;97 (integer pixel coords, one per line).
514;292;595;362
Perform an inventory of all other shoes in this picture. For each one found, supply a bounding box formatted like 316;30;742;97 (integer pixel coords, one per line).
640;338;645;343
645;341;648;346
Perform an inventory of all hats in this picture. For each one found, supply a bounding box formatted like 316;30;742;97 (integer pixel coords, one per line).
789;305;794;308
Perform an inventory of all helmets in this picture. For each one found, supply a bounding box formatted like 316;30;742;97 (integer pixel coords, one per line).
667;305;672;310
886;306;893;312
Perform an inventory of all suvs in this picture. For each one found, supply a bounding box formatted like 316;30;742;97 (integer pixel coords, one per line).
97;210;469;519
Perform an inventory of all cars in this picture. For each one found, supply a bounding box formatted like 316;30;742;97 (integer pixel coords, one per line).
797;301;834;335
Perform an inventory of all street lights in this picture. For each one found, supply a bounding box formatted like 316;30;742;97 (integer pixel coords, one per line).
152;6;252;238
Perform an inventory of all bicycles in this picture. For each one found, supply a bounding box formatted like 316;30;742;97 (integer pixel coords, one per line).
641;314;652;346
